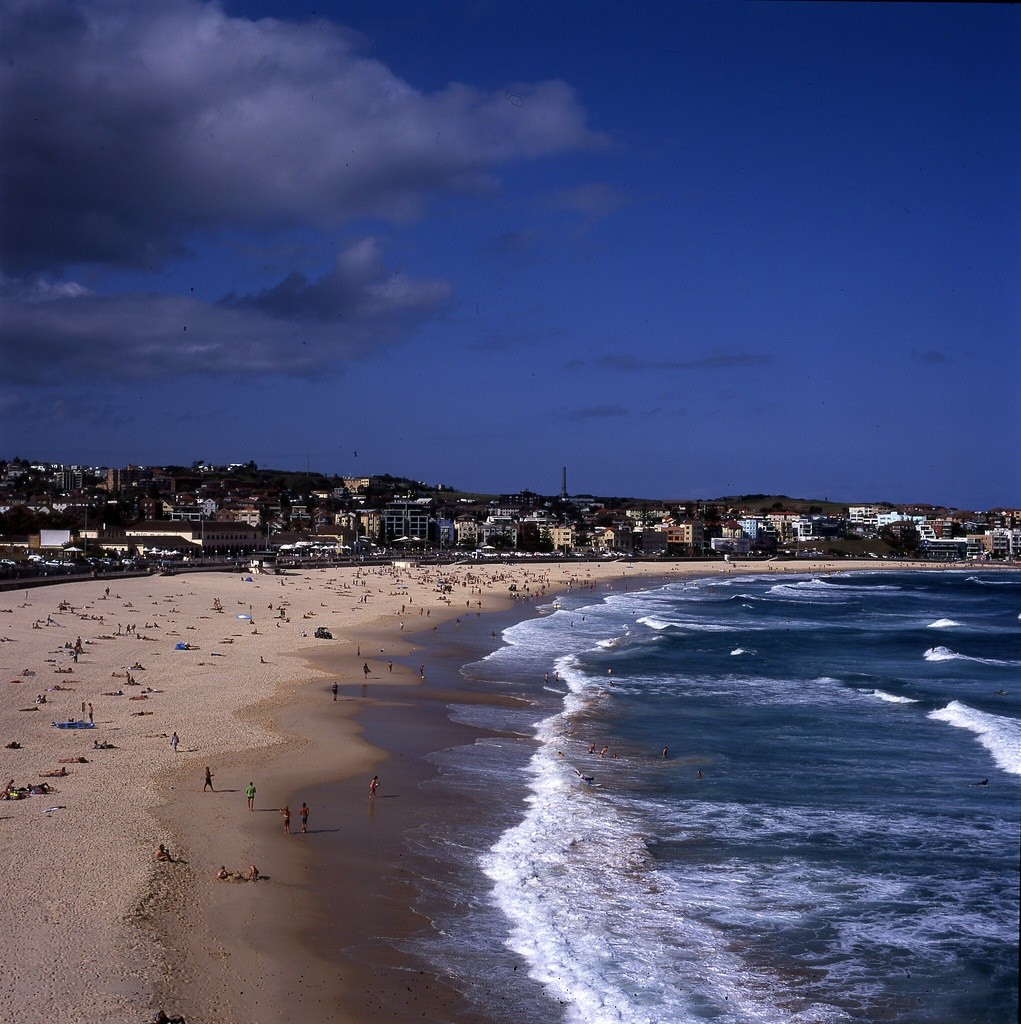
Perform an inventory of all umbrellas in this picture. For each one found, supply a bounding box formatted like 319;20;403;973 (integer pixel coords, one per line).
143;546;182;557
311;545;351;553
63;546;84;551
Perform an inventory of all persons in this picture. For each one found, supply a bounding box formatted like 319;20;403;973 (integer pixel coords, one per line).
0;560;1020;882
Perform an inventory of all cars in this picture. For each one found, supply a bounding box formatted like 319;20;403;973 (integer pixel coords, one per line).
0;554;171;568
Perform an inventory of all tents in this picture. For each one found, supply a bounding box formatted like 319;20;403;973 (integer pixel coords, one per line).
280;544;294;556
483;545;495;554
